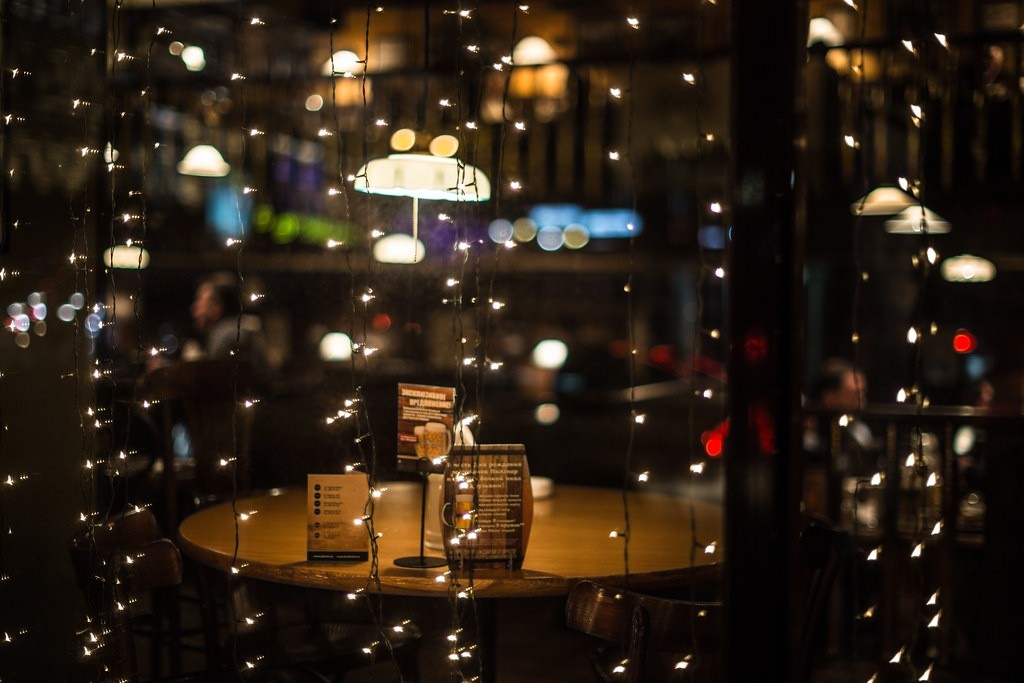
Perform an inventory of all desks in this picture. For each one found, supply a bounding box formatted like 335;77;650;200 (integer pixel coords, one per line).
176;482;723;683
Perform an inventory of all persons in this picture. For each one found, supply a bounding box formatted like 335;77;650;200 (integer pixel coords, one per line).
192;282;261;359
805;358;873;450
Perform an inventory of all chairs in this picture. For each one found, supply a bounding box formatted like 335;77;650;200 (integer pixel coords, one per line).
69;482;850;683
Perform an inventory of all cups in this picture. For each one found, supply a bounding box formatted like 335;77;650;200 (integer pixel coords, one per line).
424;423;452;459
425;474;444;547
413;426;426;458
